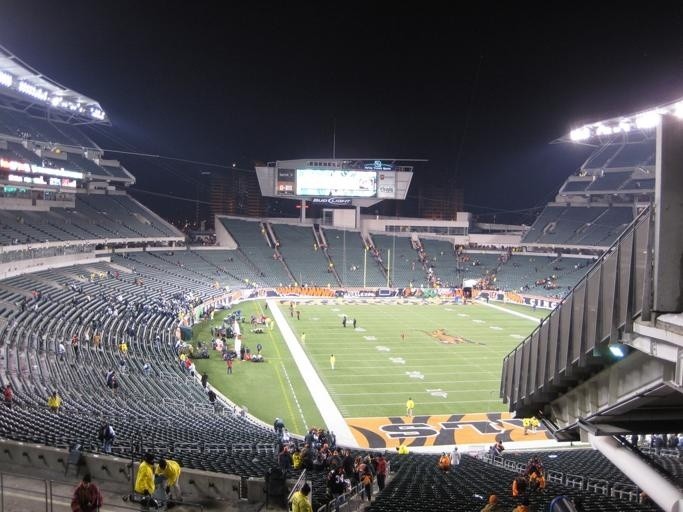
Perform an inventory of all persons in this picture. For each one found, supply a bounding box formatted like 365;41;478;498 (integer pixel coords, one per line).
0;236;683;512
